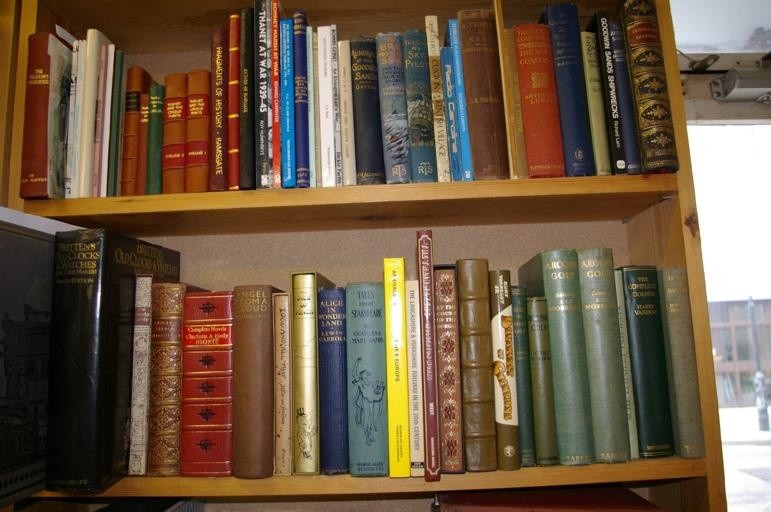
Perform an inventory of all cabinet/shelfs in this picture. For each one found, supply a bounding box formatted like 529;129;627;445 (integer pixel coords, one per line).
5;1;733;512
